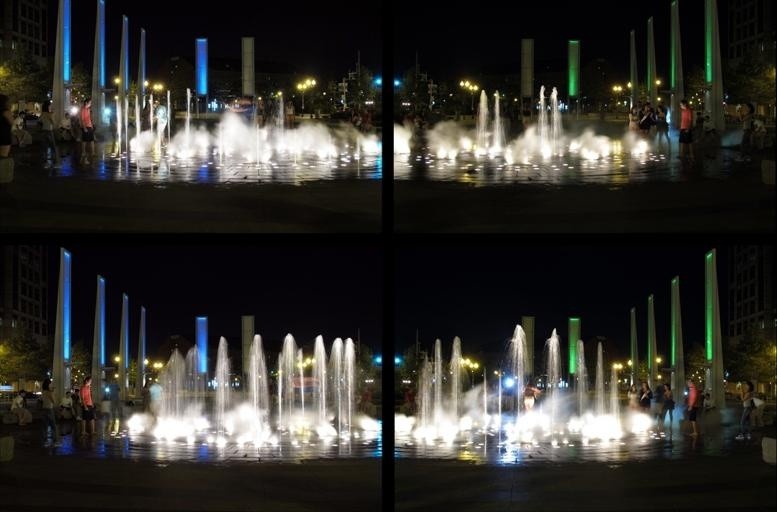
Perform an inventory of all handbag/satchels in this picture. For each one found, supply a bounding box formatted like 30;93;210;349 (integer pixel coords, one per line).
751;121;754;131
751;399;756;409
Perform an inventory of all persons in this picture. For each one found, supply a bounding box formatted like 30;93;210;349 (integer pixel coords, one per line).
522;383;543;411
351;107;369;130
0;93;123;170
629;99;768;161
230;100;296;130
735;381;767;440
141;98;170;148
10;376;126;449
138;380;152;411
627;376;716;438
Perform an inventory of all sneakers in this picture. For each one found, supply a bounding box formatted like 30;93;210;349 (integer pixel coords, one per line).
677;156;695;162
735;434;751;440
81;432;96;435
735;155;751;161
685;432;698;436
43;442;63;447
